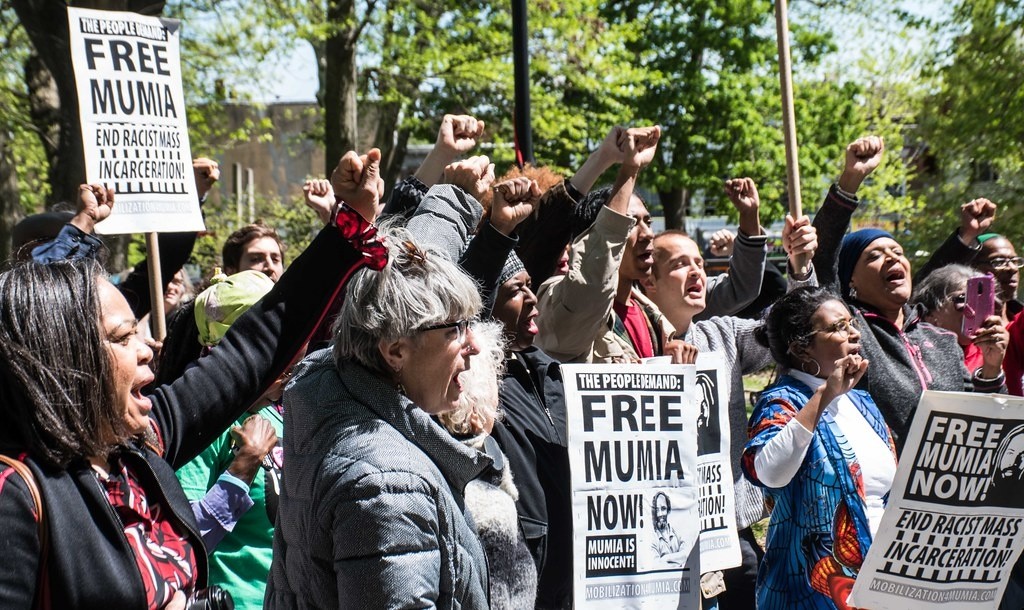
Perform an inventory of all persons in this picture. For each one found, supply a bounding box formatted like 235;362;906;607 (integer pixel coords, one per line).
263;153;497;610
0;147;384;610
1;112;1023;610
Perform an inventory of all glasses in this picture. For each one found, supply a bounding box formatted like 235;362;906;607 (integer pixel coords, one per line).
948;292;966;311
786;318;861;353
411;316;474;344
976;257;1024;270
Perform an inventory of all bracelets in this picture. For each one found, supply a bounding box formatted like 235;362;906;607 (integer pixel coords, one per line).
835;176;856;197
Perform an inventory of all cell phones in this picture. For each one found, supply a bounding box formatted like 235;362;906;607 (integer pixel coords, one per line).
961;276;994;340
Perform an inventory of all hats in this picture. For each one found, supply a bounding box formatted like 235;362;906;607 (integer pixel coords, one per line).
459;234;526;288
838;227;895;292
11;210;97;255
977;233;1000;244
194;269;275;347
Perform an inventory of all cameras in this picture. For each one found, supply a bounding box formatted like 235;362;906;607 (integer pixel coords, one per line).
180;584;234;610
